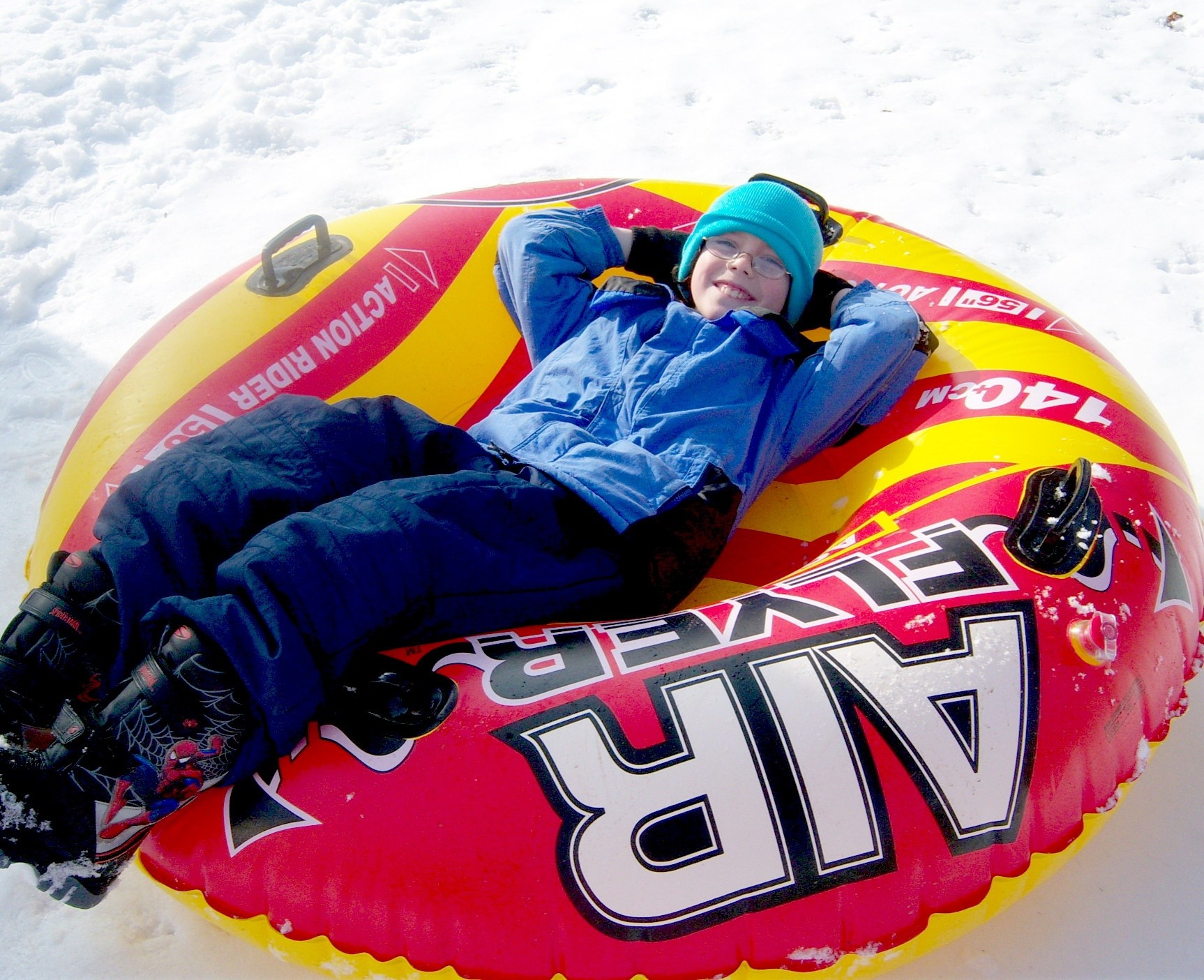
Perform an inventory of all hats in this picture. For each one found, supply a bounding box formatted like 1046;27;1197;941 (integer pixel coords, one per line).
679;181;824;329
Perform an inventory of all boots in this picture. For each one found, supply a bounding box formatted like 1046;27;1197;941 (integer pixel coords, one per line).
0;549;122;753
0;623;249;909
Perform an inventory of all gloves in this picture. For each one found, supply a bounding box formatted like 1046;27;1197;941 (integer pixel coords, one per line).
625;226;689;297
798;268;852;330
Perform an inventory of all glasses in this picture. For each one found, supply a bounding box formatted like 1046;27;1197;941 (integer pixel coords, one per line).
703;235;797;280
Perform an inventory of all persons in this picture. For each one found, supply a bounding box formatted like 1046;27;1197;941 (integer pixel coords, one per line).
0;173;920;909
